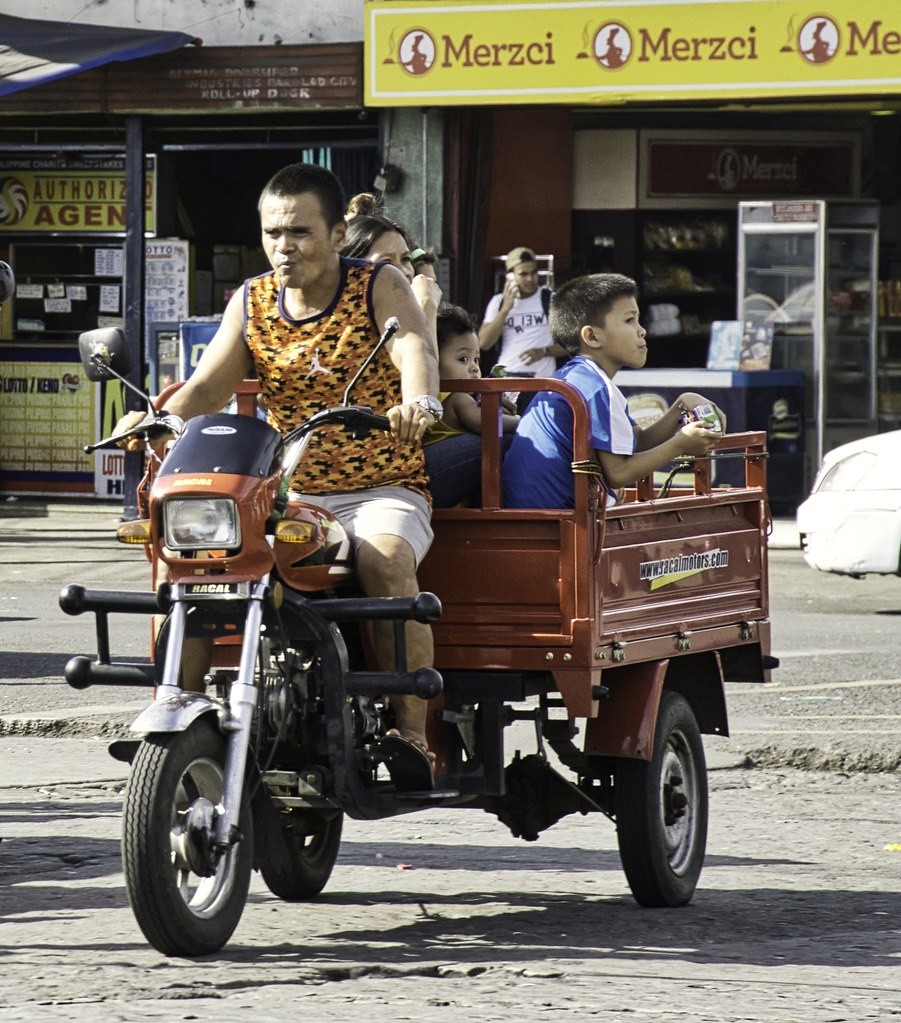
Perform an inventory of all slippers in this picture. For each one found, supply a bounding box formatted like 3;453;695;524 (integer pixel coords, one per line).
379;736;436;790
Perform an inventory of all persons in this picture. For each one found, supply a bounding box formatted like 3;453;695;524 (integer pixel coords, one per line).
339;192;726;509
112;165;444;793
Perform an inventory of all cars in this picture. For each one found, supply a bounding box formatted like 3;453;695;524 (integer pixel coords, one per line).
750;268;901;407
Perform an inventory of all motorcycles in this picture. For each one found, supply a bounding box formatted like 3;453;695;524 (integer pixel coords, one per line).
57;327;778;956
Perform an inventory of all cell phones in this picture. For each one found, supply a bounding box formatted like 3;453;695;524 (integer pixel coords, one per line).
505;272;521;298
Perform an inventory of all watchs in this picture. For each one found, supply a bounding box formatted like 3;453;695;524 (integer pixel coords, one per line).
543;346;550;358
409;395;443;423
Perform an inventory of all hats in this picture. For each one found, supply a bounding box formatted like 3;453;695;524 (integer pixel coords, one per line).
505;247;537;271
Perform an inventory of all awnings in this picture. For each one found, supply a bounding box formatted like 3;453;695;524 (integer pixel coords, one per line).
0;14;204;96
